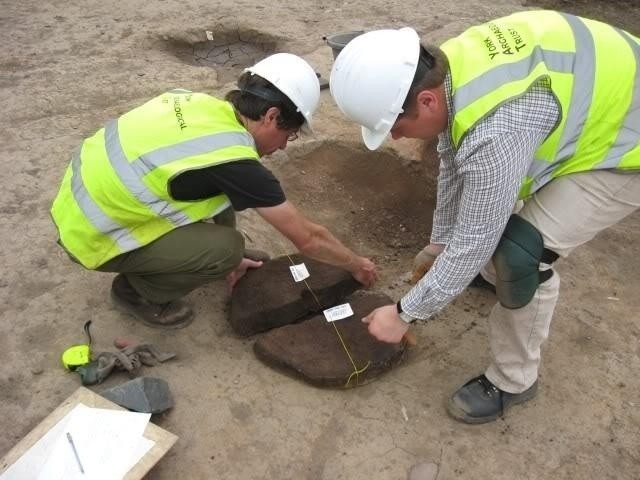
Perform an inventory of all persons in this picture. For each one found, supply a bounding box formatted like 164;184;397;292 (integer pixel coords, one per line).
49;51;382;332
328;8;640;424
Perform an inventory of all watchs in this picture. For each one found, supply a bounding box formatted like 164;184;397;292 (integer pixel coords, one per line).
396;300;417;325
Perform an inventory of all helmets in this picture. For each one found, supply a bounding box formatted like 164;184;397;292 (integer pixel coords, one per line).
330;27;419;151
242;52;320;133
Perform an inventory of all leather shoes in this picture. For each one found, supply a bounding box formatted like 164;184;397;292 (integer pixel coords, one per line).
469;273;497;294
243;249;269;261
110;275;194;330
447;374;538;424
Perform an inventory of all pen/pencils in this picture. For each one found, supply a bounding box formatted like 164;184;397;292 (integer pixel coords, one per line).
66;433;85;473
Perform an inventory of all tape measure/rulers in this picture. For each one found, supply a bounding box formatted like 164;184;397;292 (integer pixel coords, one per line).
62;320;92;371
